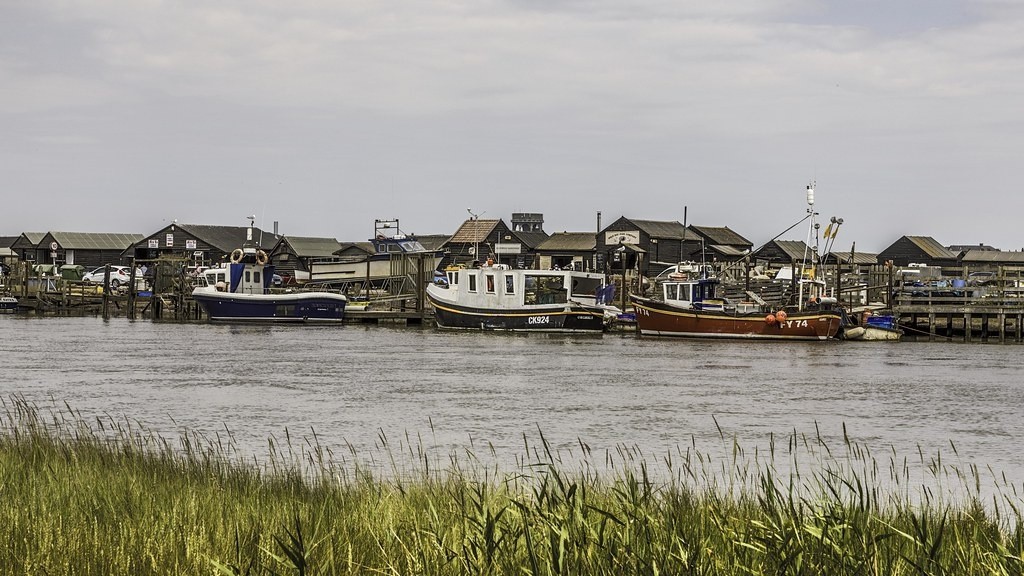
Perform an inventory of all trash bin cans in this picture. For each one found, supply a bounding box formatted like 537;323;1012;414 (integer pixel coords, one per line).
60;265;84;280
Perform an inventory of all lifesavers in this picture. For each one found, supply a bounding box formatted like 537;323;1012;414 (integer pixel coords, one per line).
230;248;243;264
256;250;267;265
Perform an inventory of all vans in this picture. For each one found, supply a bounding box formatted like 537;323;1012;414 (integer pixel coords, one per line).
655;263;716;281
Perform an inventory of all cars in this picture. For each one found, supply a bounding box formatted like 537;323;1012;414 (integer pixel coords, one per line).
82;265;144;289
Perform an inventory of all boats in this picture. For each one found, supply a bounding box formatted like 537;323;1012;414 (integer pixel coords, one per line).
425;256;606;335
0;296;18;314
626;184;842;342
346;295;370;312
842;314;906;341
192;214;347;327
